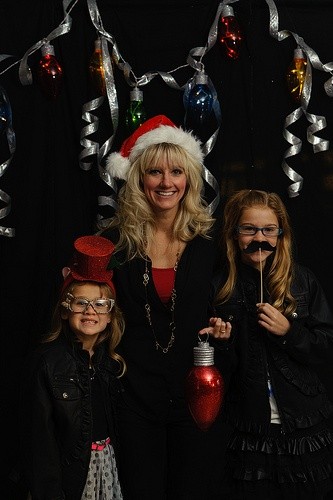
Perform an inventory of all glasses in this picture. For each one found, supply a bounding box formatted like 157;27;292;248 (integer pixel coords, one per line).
61;292;115;314
236;224;283;236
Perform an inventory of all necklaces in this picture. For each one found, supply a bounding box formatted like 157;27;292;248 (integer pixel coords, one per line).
143;214;180;353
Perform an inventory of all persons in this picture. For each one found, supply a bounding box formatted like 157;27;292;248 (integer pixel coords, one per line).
200;189;333;500
24;235;126;500
104;114;226;500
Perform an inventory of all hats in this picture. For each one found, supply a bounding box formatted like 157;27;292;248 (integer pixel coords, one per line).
62;235;115;297
105;114;203;181
